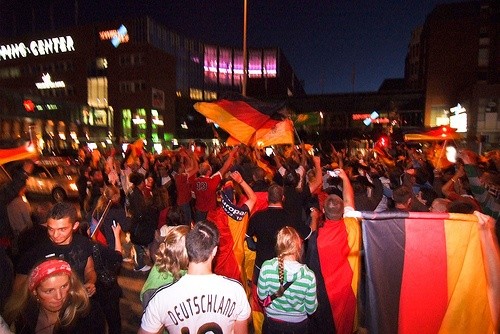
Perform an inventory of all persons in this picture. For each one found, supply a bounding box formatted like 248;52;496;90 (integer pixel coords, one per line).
255;225;320;334
0;113;500;228
8;256;110;334
90;186;136;253
316;168;363;334
16;209;51;254
278;156;324;266
121;182;142;265
245;186;319;334
0;202;98;304
206;171;257;301
149;205;193;264
138;225;187;334
71;203;126;334
124;172;153;273
138;220;253;334
4;178;33;238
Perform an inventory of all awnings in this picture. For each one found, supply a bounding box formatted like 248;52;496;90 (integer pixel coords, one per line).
403;128;467;142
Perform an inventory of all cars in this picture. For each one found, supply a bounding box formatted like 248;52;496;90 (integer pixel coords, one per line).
25;156;82;204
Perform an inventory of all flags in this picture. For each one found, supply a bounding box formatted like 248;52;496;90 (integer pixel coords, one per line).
225;118;293;147
360;209;500;334
0;144;38;166
192;99;278;146
